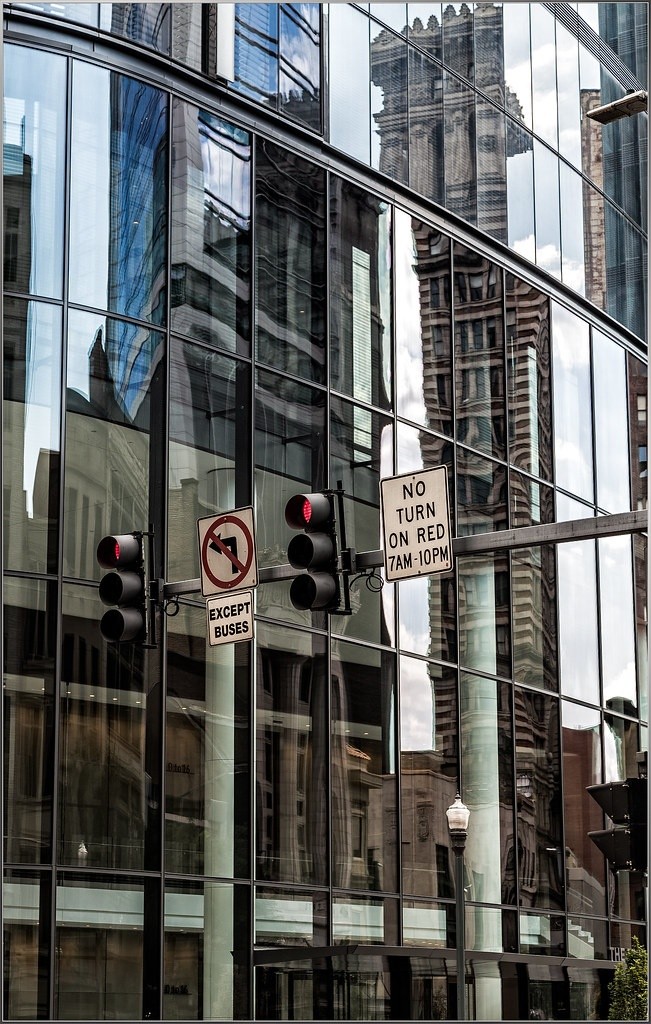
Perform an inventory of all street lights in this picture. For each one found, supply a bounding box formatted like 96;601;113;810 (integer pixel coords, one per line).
445;789;470;1020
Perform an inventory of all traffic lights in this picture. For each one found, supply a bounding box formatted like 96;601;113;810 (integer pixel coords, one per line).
285;493;342;613
96;530;147;645
584;776;648;873
